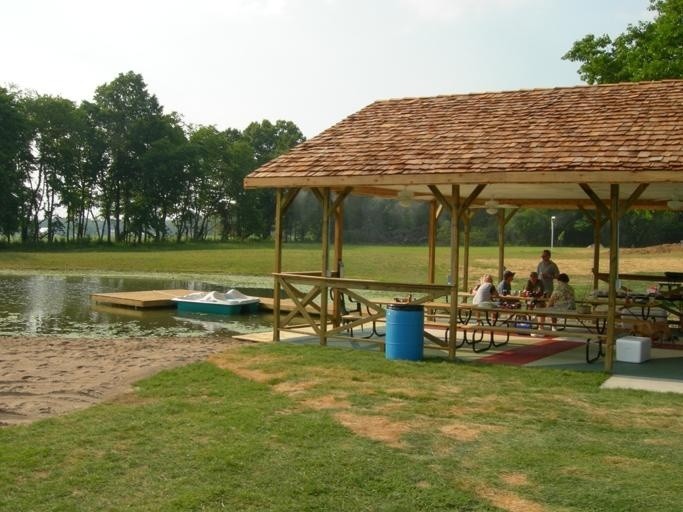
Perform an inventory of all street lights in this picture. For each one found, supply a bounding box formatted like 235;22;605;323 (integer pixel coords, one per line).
550;215;558;260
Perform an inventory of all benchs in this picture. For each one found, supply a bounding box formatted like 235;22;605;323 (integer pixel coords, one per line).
339;291;683;365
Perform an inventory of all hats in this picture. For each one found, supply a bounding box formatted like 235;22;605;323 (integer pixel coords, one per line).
503;270;516;279
555;273;569;283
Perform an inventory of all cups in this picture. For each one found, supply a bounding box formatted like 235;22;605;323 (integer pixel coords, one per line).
503;290;507;296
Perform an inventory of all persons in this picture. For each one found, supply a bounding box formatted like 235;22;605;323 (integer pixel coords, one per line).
536;250;561;332
472;270;576;338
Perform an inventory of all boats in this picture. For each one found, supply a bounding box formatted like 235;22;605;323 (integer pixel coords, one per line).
171;284;262;317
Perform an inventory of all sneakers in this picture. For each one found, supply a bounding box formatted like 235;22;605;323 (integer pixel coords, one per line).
530;329;546;338
552;325;557;332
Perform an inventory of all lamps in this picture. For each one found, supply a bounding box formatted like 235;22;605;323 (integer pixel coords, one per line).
484;184;499;215
666;194;683;212
397;185;414;208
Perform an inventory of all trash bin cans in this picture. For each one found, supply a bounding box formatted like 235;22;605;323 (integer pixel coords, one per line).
385;304;424;361
